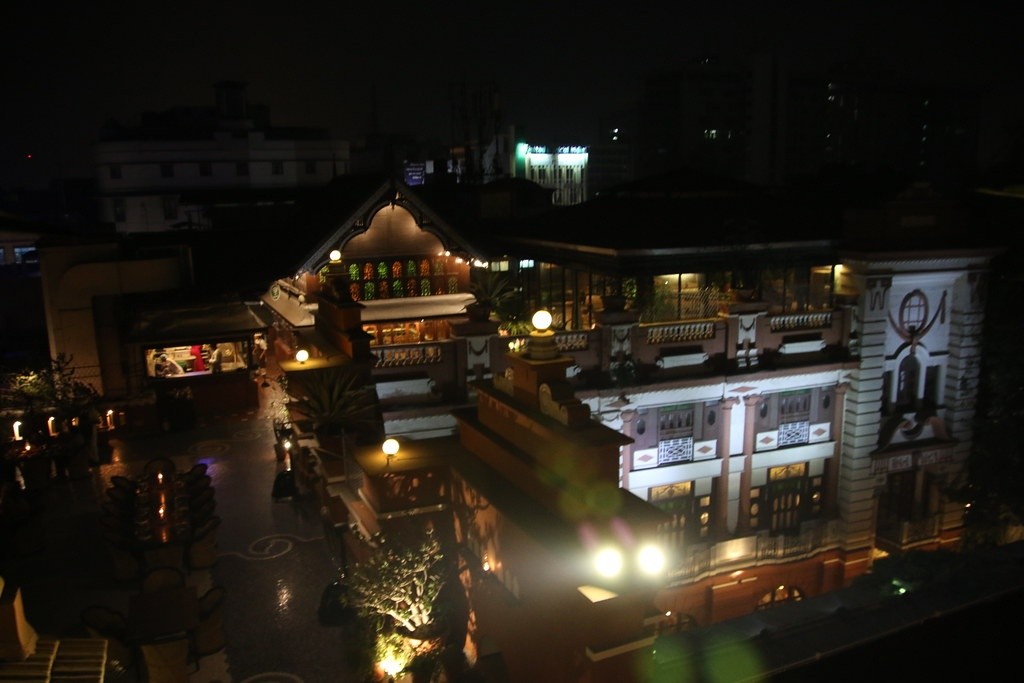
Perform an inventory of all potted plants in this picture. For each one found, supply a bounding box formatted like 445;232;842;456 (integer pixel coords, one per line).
277;363;389;452
462;263;512;320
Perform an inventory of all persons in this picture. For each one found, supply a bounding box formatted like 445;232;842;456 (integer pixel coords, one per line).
608;356;621;384
624;353;637;379
79;402;103;482
294;447;323;504
